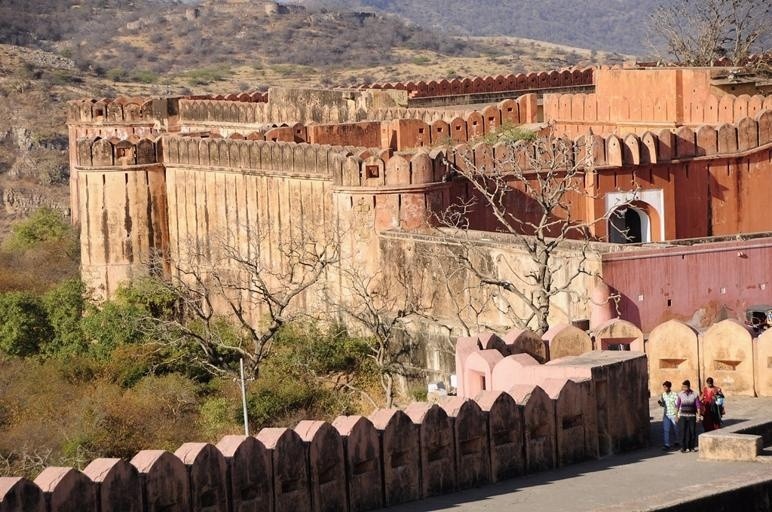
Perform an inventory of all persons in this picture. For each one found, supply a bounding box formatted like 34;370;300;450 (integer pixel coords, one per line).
674;379;701;452
656;381;682;451
698;376;725;432
